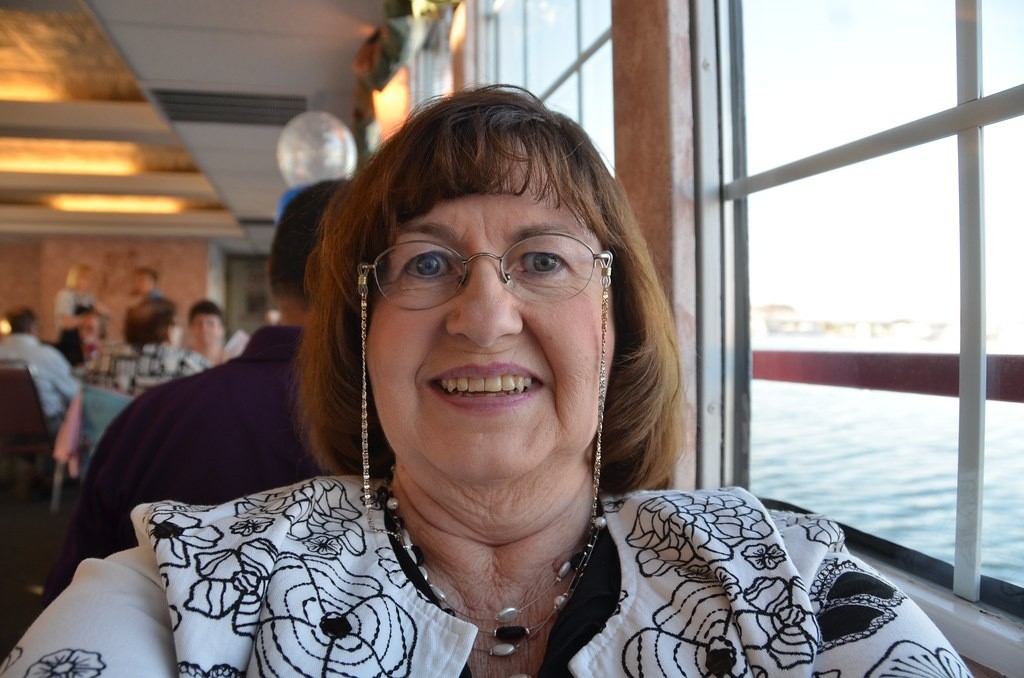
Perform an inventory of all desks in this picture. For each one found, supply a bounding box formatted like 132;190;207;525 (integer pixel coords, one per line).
68;362;187;479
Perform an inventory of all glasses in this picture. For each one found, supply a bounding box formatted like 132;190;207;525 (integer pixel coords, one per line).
359;232;612;311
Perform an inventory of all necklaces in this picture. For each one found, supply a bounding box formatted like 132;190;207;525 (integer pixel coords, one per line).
377;465;607;658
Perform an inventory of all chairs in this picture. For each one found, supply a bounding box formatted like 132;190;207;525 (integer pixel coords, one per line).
0;365;91;456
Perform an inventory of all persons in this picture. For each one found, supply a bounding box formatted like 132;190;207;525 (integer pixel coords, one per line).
0;86;974;678
0;264;228;499
44;180;388;603
